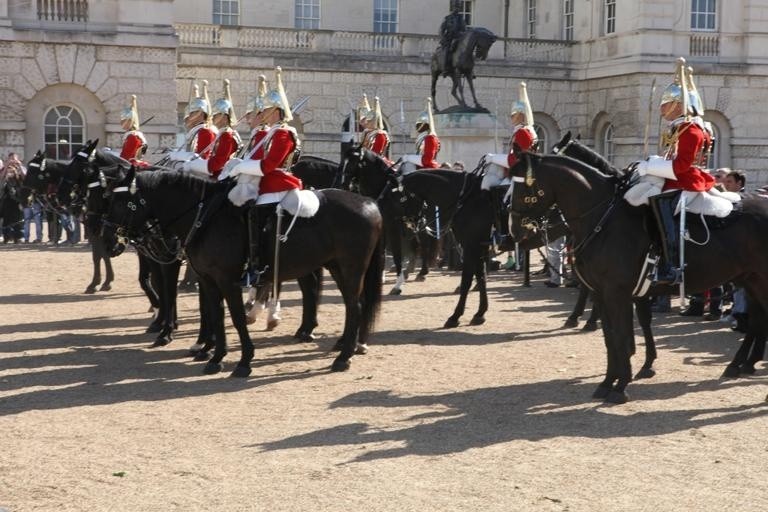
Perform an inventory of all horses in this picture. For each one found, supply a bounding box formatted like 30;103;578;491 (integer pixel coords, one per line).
430;27;497;113
399;168;607;335
552;131;657;381
20;149;117;294
290;155;341;190
330;136;442;296
137;254;283;334
56;138;175;208
98;165;384;379
508;141;766;406
137;166;218;363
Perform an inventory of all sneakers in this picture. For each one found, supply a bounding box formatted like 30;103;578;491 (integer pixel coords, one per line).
4;238;77;245
543;279;578;288
652;302;721;320
721;315;738;326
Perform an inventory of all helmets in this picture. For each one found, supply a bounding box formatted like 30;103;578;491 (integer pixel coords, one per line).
183;79;237;126
119;95;139;131
660;57;704;116
360;93;384;129
510;81;534;126
416;96;434;135
245;66;292;123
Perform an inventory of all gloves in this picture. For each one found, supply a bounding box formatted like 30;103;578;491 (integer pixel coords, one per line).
639;158;678;181
230;158;264;178
403;154;423;168
170;151;198;162
183;158;214;177
485;153;509;169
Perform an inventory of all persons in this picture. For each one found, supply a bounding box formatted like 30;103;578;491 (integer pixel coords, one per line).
1;94;148;246
439;161;464;270
484;81;539;168
636;57;751;324
169;65;301;283
440;1;466;77
359;92;441;170
544;236;579;287
